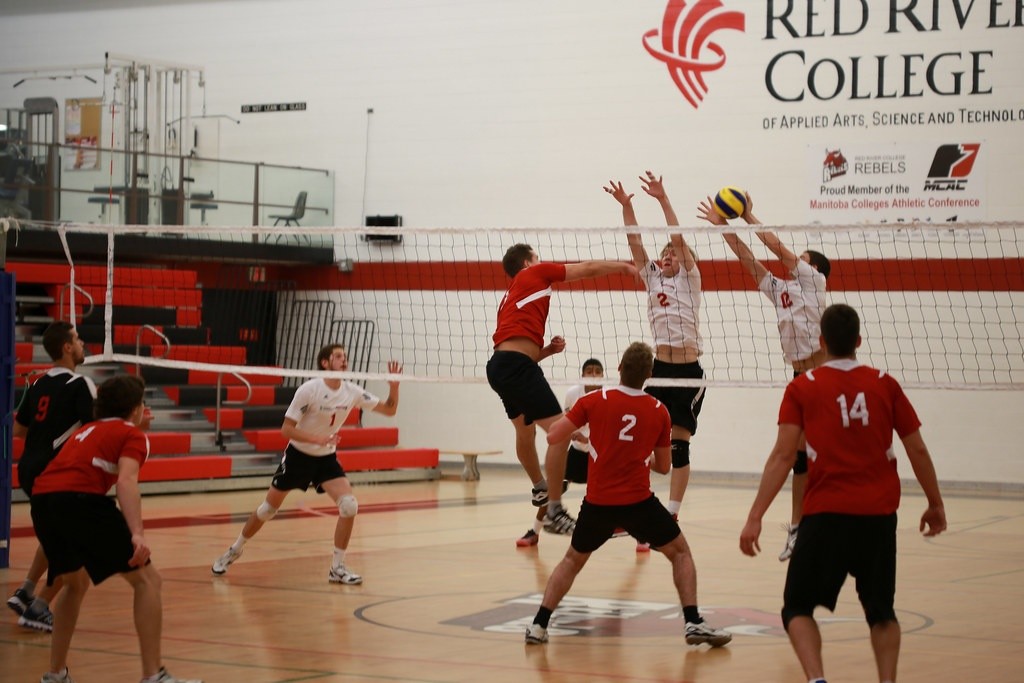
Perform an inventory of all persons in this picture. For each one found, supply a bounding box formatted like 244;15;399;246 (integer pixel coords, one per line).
524;343;732;648
696;189;831;563
516;358;651;553
6;319;97;632
30;375;206;683
212;344;403;585
740;305;947;683
485;244;640;536
604;169;706;539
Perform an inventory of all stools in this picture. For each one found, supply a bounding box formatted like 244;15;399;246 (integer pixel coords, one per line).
87;197;120;225
190;203;219;224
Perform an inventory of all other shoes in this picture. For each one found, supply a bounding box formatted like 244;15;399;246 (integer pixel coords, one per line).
141;667;203;683
40;666;76;683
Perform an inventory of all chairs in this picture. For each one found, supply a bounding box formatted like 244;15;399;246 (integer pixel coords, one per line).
265;191;310;246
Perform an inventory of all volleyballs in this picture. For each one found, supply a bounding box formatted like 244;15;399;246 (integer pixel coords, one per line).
714;187;747;219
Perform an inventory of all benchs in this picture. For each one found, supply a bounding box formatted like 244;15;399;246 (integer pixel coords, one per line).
5;263;442;504
437;449;505;483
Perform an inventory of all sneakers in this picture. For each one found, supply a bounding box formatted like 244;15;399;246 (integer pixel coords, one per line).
636;538;652;551
525;623;550;644
542;511;576;535
611;528;631;537
212;547;245;574
778;521;799;562
18;606;54;631
516;530;538;546
532;481;569;507
7;589;35;614
684;617;732;647
329;565;363;584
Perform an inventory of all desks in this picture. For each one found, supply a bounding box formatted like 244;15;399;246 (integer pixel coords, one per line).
92;185;215;228
